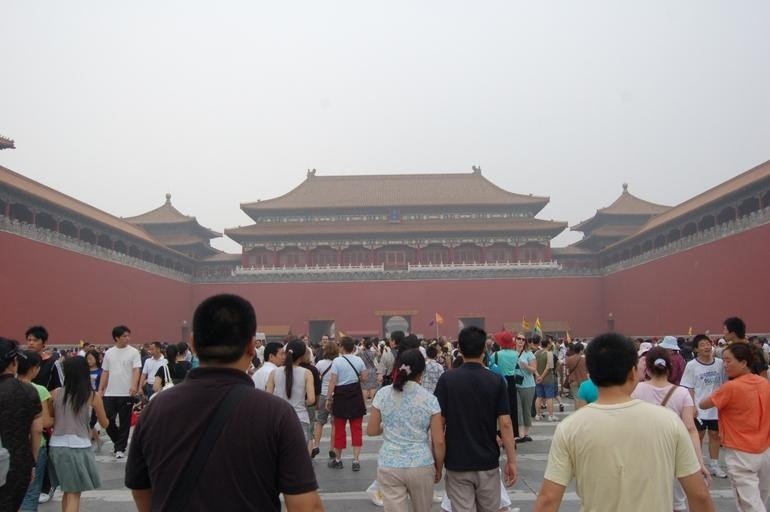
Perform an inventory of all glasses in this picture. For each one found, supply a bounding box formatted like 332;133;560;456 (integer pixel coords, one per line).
517;334;526;343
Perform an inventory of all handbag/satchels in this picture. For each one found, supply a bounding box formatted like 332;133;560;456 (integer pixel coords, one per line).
147;364;176;402
562;373;572;391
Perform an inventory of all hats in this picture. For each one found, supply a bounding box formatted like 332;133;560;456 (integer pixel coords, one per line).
662;334;680;351
497;330;515;347
638;342;654;357
716;337;728;348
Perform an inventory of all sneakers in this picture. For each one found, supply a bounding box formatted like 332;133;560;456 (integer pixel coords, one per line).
557;403;567;413
327;447;339;458
114;450;126;460
707;457;730;480
349;458;362;474
512;433;538;446
547;413;560;424
534;412;545;424
37;492;54;508
326;459;346;471
309;446;321;460
